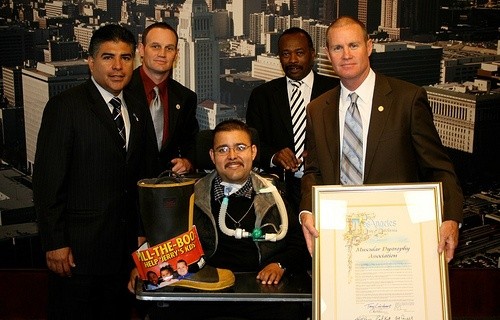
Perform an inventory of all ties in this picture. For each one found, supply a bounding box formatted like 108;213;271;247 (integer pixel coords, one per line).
109;97;126;154
149;86;164;154
339;92;363;186
289;81;307;172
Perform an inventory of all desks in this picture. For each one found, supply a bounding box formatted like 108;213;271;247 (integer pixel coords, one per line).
135;269;312;303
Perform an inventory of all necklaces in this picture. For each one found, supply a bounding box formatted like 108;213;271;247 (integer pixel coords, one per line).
217;196;253;229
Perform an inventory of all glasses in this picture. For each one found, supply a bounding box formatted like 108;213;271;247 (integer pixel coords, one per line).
212;145;251;155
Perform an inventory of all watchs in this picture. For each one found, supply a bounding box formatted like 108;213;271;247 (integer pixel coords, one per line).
277;263;285;270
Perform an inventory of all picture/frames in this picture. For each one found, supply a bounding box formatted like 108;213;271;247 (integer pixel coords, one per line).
312;181;452;320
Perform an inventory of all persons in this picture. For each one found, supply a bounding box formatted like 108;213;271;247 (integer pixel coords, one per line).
125;22;201;177
33;24;144;320
247;27;340;222
146;260;195;290
298;15;463;262
127;120;300;320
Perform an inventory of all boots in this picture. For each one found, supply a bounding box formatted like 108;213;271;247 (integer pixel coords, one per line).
136;171;235;290
